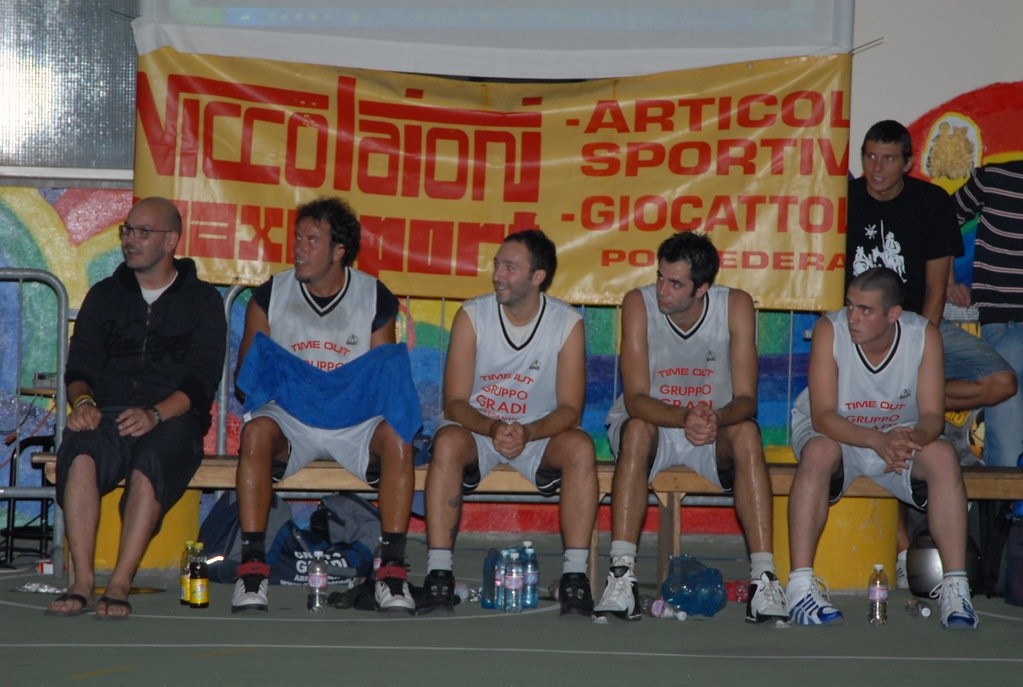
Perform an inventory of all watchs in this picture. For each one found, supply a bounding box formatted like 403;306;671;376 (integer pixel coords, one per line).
145;405;162;424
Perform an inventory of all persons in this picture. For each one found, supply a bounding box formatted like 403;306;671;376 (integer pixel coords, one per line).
43;197;229;622
785;264;981;631
592;230;792;628
945;159;1023;468
230;196;417;614
422;225;599;625
842;119;1019;413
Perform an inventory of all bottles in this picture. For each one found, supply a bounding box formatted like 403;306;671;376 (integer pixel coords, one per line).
637;594;687;623
547;579;560;602
372;536;383;573
903;597;932;620
179;540;210;609
480;540;541;612
306;551;329;613
866;563;889;626
452;579;483;603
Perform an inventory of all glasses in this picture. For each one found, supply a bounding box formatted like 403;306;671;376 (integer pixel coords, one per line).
119;225;172;239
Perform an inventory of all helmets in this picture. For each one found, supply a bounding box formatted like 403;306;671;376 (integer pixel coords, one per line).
907;528;984;597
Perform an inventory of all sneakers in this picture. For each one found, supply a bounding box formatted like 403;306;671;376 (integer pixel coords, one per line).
744;570;790;624
557;571;594;619
376;561;416;613
784;575;844;625
896;558;908;588
594;564;642;620
231;559;269;614
422;569;456;606
929;578;979;629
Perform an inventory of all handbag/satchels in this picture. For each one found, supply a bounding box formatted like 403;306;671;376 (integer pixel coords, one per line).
197;487;381;588
985;503;1022;607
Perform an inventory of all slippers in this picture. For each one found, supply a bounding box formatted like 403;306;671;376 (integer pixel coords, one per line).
44;592;94;617
95;595;130;621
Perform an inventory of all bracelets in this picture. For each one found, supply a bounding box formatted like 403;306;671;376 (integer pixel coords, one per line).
489;419;503;438
71;394;97;409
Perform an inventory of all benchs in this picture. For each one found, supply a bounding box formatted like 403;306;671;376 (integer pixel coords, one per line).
30;453;1023;611
